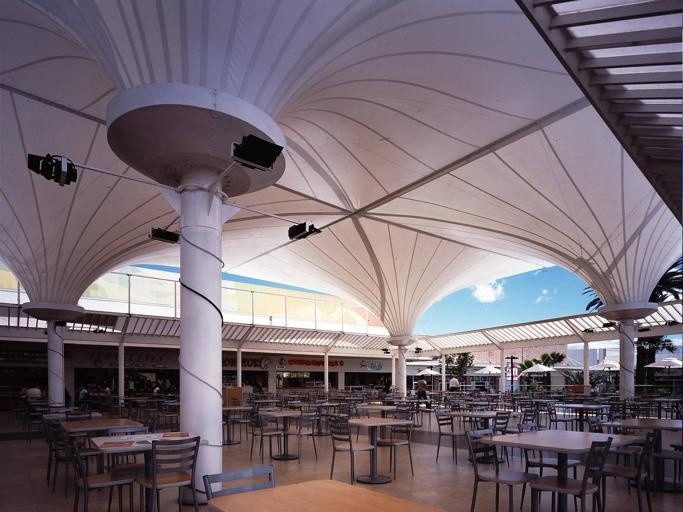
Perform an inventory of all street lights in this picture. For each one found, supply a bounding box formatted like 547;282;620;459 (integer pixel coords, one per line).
505;355;520;400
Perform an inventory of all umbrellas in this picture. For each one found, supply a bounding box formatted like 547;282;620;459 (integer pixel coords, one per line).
588;361;620;392
553;356;584;374
414;369;440;378
520;363;556;384
474;365;507;374
643;356;682;395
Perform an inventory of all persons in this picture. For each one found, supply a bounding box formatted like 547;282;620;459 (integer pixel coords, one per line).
414;375;429;409
18;377;166;411
447;374;459;392
